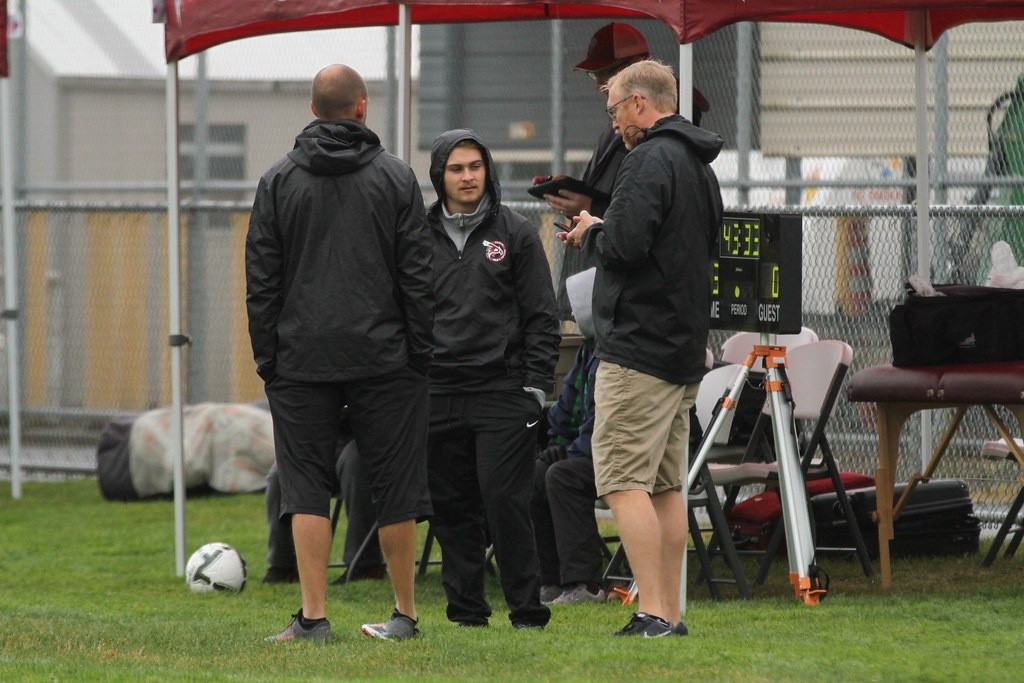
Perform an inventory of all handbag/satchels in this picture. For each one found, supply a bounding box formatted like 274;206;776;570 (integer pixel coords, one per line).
889;281;1024;367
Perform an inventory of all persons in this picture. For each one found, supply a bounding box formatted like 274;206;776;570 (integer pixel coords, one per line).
425;129;561;632
256;402;388;583
532;19;726;638
245;64;439;644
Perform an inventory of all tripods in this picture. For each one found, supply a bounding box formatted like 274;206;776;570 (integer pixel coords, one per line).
613;333;827;604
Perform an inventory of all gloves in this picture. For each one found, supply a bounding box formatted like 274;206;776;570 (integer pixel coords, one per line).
539;444;582;466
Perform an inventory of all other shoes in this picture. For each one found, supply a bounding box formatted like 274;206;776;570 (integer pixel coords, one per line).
329;567;388;584
262;567;300;584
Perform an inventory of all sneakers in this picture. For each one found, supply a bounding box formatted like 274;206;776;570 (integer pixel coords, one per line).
361;608;418;644
672;621;688;636
540;583;605;604
613;612;673;638
264;608;331;642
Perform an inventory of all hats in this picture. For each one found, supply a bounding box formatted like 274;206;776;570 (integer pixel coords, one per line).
572;22;649;72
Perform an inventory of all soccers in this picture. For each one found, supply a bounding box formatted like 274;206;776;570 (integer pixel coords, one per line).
183;542;246;600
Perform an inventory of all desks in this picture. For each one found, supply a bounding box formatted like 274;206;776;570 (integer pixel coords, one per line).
844;360;1024;589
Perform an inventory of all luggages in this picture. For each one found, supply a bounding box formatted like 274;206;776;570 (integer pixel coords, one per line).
810;479;981;562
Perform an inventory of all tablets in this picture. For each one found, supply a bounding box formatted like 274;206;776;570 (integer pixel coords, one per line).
528;174;611;205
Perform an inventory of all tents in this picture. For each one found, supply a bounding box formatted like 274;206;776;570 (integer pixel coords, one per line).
0;1;27;505
151;0;1024;588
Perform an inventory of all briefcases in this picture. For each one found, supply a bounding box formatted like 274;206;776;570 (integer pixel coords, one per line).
723;473;875;555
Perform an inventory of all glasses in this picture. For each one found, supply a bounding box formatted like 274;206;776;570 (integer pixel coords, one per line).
586;57;633;83
605;95;645;121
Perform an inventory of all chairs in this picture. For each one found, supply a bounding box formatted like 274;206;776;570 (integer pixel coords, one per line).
328;327;875;598
